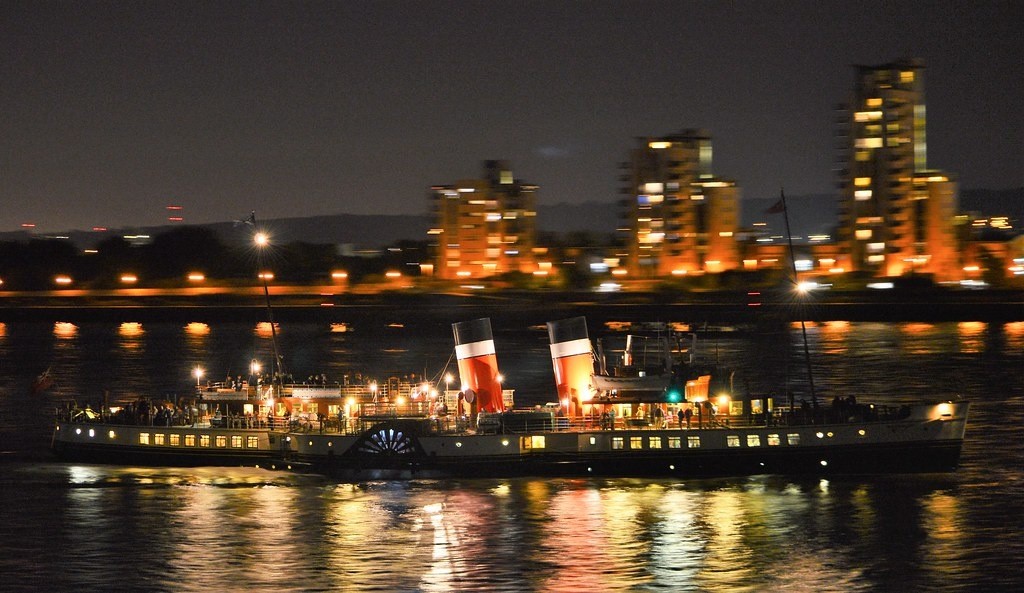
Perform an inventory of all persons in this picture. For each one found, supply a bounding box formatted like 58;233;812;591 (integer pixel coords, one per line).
832;394;856;408
655;406;664;430
710;403;718;414
308;373;326;385
207;381;212;392
799;398;810;415
558;407;564;430
227;372;292;391
678;407;693;429
337;406;343;433
215;408;220;415
636;407;645;419
436;401;449;430
344;371;416;387
125;396;190;425
602;408;615;430
230;407;299;430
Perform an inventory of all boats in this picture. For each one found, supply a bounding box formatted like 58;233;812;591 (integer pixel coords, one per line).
45;184;975;478
